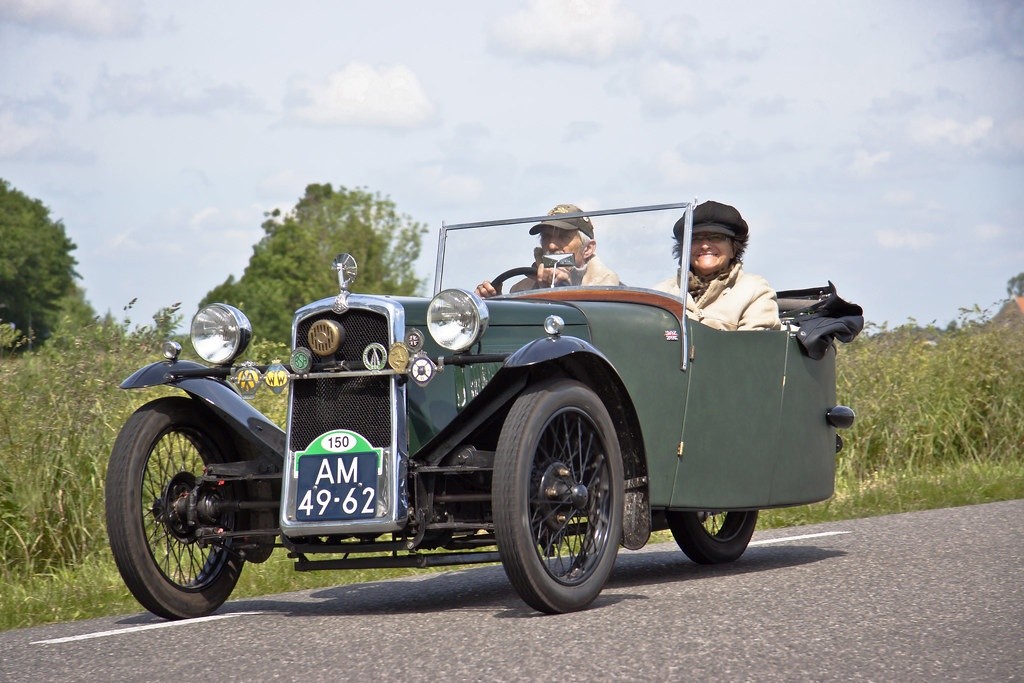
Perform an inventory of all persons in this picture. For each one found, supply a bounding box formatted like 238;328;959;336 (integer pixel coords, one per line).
651;200;782;332
473;204;619;300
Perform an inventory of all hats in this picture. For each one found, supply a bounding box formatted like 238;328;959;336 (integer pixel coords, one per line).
529;204;594;239
673;200;747;243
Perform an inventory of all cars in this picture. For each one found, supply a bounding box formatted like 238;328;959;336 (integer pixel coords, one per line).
105;199;864;620
